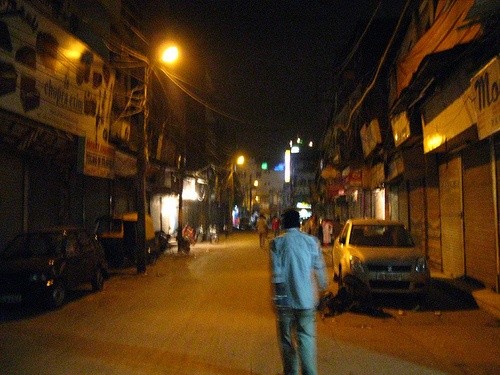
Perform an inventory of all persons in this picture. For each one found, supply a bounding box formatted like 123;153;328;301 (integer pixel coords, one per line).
256;213;281;247
269;209;328;375
183;223;219;247
300;213;345;247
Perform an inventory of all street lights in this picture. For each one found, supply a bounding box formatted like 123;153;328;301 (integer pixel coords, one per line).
142;44;179;261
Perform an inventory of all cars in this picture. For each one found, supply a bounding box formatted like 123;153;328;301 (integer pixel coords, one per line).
331;217;430;301
0;225;111;309
